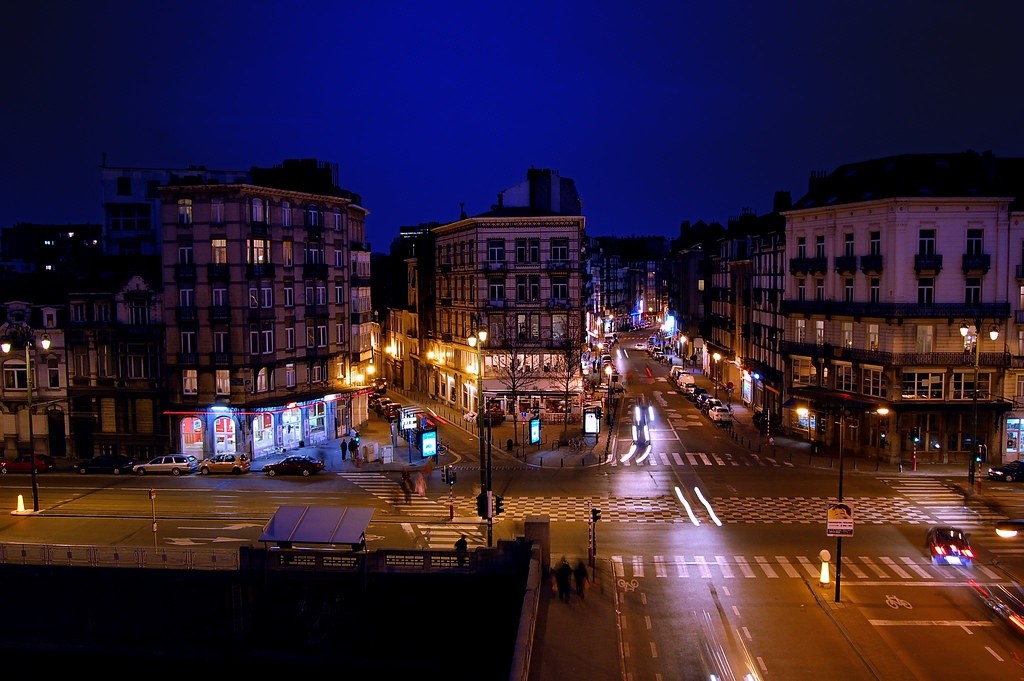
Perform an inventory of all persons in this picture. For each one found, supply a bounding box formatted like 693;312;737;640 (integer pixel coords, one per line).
401;464;427;504
348;438;356;462
554;560;589;604
454;535;467;566
340;439;347;461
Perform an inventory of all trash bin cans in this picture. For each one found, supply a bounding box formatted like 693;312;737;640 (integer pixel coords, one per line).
507;438;513;451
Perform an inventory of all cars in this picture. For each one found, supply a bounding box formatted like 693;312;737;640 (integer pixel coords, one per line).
71;453;134;475
986;459;1024;483
925;524;974;565
0;453;55;475
261;454;326;477
995;518;1024;540
132;452;199;476
599;319;732;424
367;377;403;424
197;451;251;476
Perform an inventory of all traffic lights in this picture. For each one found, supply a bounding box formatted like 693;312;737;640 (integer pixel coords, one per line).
440;465;447;484
912;427;919;443
496;494;505;515
592;509;601;522
450;473;456;485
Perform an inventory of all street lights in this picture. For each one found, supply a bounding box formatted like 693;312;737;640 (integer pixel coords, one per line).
0;321;52;511
712;353;721;399
465;313;488;519
605;365;613;426
875;407;889;471
679;335;686;370
956;302;1000;486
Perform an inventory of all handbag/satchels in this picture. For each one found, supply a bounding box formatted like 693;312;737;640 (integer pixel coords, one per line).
585;580;589;590
552;576;558;592
